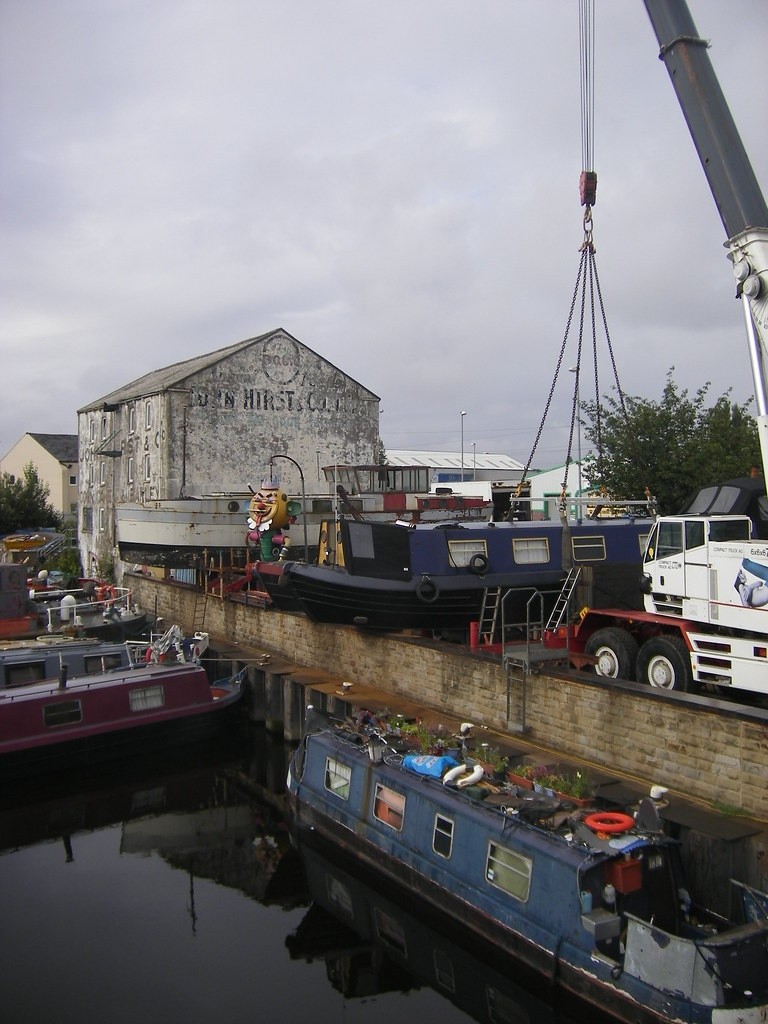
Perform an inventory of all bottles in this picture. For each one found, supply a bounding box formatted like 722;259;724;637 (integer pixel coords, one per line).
605;883;616;904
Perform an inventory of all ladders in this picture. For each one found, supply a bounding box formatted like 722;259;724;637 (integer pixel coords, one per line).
189;590;209;633
538;566;583;640
504;658;528;735
476;586;502;645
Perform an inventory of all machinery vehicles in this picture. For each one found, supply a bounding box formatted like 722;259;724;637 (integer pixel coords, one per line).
542;0;768;699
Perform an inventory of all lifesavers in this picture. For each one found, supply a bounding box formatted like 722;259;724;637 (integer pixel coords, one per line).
442;762;484;792
145;646;167;665
96;585;117;607
583;811;636;833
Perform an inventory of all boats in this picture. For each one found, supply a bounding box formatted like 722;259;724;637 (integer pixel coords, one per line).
1;563;149;642
273;815;622;1024
1;638;247;770
281;515;657;642
0;531;66;568
115;466;495;569
288;717;768;1024
253;560;300;611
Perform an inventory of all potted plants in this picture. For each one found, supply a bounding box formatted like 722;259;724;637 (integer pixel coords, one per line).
374;708;597;808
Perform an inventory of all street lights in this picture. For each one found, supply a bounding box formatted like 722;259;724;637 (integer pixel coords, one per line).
459;410;467;482
471;442;475;481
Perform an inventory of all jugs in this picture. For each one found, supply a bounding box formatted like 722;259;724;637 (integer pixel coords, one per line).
578;891;593;913
